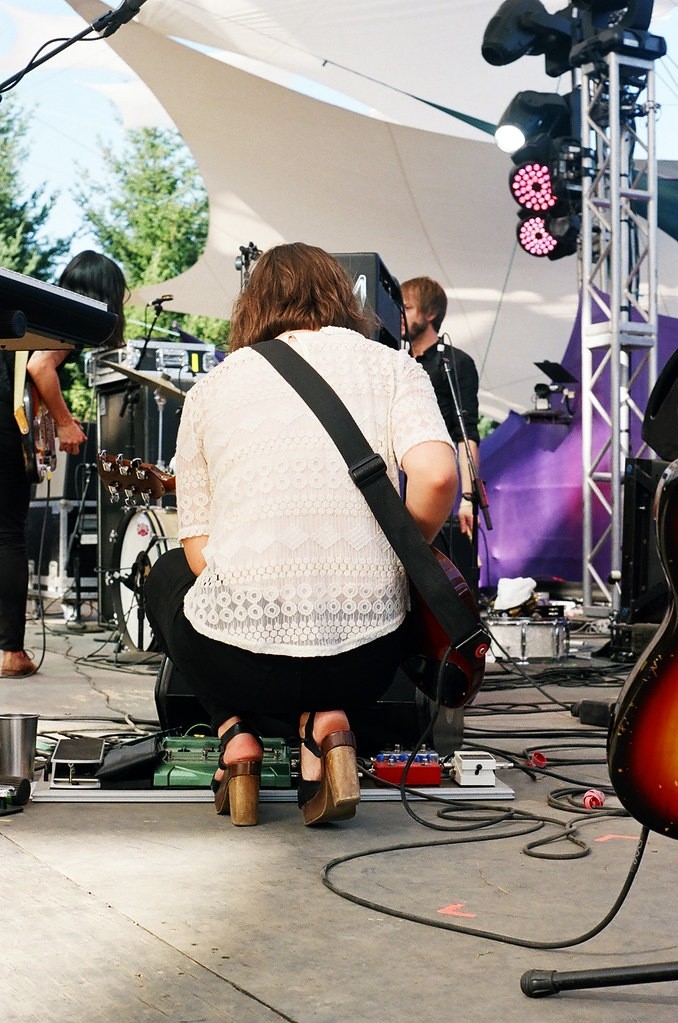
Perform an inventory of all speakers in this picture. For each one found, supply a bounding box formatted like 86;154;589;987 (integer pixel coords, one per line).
93;381;200;630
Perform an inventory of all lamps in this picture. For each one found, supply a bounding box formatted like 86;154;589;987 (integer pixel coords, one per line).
516;208;582;262
509;133;564;213
495;89;569;154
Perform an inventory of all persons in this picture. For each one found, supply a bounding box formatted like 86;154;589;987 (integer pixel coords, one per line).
390;272;488;604
134;239;458;828
1;245;131;685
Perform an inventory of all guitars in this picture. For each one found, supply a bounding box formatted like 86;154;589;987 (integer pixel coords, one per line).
605;451;678;845
19;382;57;483
87;450;485;712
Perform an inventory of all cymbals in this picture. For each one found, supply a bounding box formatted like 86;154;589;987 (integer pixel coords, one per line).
101;357;186;401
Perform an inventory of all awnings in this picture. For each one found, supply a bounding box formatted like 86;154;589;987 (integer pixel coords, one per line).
48;0;677;482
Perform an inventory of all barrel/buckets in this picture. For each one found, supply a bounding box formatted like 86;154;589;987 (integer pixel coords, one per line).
1;711;41;782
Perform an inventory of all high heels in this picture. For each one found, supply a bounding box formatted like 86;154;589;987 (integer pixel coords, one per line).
297;710;361;826
210;721;264;826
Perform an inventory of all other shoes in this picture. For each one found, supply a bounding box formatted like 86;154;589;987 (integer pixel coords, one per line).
2;651;38;677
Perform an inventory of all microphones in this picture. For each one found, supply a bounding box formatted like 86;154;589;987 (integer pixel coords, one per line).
81;462;97;472
431;338;445;384
147;295;173;305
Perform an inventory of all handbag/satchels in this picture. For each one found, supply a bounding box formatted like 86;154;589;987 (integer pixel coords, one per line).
19;380;57;485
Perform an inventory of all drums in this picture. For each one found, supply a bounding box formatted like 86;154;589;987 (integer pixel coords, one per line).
108;498;179;651
